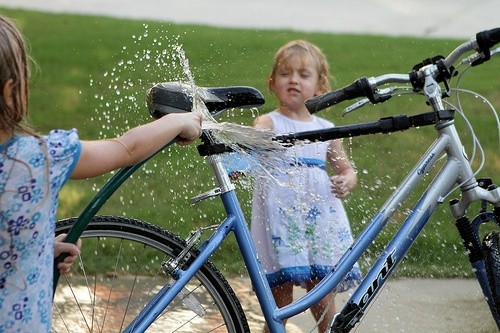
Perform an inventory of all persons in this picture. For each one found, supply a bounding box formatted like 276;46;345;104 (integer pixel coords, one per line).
0;12;203;333
244;37;358;333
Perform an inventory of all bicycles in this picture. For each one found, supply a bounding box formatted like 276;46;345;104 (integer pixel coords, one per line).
49;24;500;332
